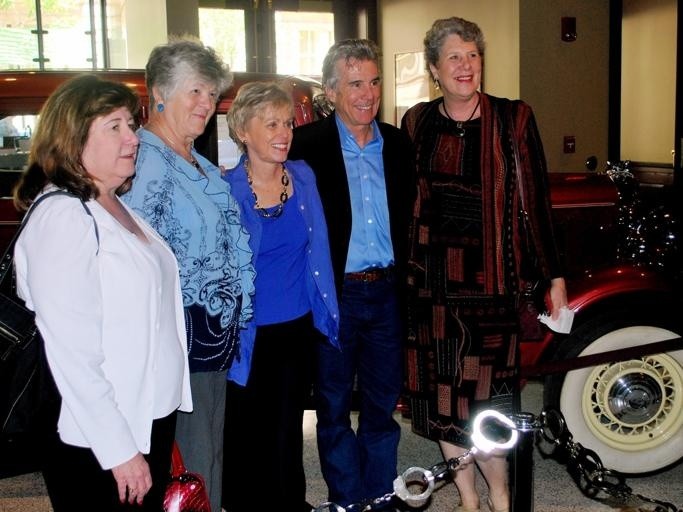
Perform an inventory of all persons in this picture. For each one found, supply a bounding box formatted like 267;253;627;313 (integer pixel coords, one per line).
285;34;425;511
119;30;257;512
11;70;194;512
400;14;571;512
219;77;339;512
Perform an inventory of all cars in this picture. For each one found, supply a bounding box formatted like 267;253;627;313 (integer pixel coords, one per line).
0;58;683;483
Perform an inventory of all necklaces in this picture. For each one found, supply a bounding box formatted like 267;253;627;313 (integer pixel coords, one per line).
157;128;202;169
439;96;481;140
242;159;289;220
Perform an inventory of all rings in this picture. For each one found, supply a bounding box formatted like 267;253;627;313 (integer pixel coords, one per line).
126;486;137;496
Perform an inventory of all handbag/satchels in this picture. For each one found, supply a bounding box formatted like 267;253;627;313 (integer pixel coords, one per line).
0;264;45;435
517;268;553;343
161;438;211;512
